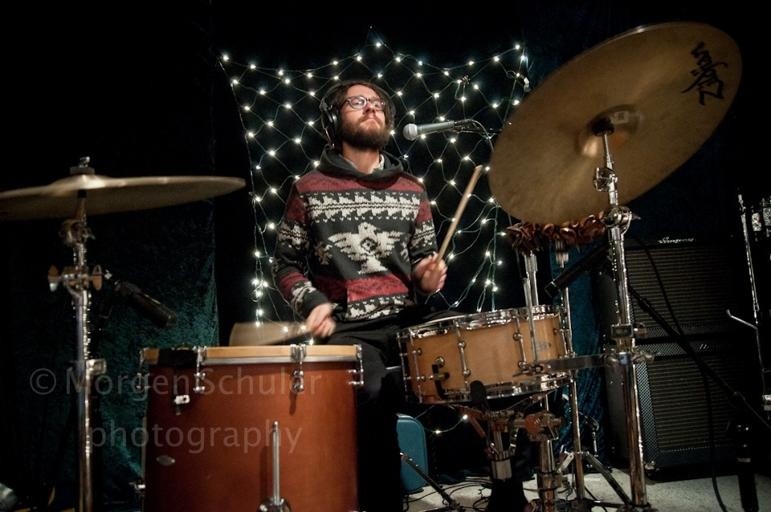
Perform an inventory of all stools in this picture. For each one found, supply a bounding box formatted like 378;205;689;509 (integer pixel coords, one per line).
382;362;466;511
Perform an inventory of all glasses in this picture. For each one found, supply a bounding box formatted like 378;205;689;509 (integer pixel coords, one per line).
338;95;386;109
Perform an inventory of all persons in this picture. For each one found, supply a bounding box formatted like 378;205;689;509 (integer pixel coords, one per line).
273;81;538;512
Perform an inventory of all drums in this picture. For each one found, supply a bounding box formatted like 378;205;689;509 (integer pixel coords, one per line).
138;343;363;511
398;303;569;405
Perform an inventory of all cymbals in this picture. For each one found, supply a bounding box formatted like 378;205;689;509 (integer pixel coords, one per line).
488;23;743;226
0;173;248;217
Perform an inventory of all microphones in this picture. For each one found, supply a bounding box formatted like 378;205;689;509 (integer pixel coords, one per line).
102;269;177;330
544;226;614;298
403;118;473;141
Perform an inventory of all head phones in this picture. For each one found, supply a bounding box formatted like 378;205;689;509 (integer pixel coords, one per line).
318;80;396;145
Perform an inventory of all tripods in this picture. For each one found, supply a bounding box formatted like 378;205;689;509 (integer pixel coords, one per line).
548;286;636;512
